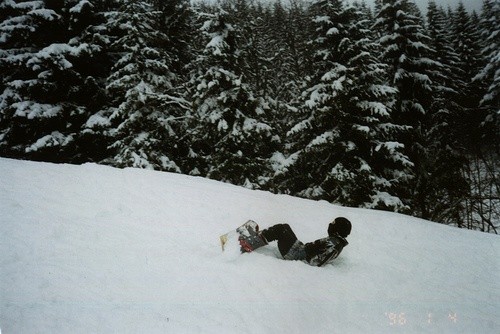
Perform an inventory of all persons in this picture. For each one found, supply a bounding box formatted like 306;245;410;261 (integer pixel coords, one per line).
239;217;351;267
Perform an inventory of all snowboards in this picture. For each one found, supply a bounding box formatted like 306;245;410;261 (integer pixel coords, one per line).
220;220;259;252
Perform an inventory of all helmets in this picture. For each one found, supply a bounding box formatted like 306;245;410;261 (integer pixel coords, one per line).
327;216;351;238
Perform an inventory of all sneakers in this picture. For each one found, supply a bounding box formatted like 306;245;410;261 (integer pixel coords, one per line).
239;235;261;252
